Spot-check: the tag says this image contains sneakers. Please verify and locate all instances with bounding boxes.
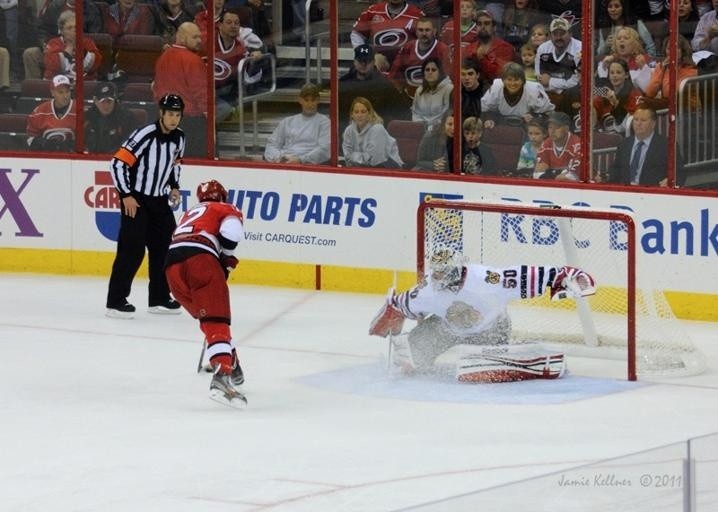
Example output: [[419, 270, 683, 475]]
[[394, 351, 432, 375], [229, 355, 245, 386], [209, 374, 247, 411]]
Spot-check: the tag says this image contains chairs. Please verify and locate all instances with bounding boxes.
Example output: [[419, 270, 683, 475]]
[[0, 33, 276, 161], [367, 45, 623, 183], [287, 0, 332, 92]]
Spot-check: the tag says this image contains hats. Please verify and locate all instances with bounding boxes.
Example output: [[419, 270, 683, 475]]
[[49, 73, 72, 90], [299, 82, 318, 99], [548, 17, 571, 32], [92, 81, 115, 103], [354, 44, 374, 60], [475, 10, 496, 20], [544, 111, 571, 127]]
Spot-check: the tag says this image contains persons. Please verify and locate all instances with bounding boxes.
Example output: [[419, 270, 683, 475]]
[[369, 251, 597, 383], [106, 94, 185, 313], [133, 0, 265, 95], [1, 0, 132, 151], [461, 1, 718, 194], [165, 180, 247, 410], [266, 1, 456, 174]]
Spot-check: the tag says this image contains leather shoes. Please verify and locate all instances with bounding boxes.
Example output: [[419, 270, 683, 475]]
[[147, 296, 180, 309], [105, 300, 135, 313]]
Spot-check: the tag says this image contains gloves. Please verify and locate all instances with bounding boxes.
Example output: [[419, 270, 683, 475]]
[[367, 288, 402, 338], [550, 261, 597, 302]]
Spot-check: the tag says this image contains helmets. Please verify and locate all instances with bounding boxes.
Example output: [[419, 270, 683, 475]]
[[196, 180, 227, 201], [158, 93, 184, 111], [426, 246, 465, 288]]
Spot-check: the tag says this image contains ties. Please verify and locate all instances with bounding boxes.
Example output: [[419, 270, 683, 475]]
[[628, 141, 644, 182]]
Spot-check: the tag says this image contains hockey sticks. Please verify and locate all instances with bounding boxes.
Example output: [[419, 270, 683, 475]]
[[197, 337, 209, 371]]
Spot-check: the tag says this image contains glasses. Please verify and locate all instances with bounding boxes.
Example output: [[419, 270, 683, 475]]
[[424, 67, 438, 72], [551, 30, 565, 35], [460, 71, 474, 76], [356, 58, 371, 64]]
